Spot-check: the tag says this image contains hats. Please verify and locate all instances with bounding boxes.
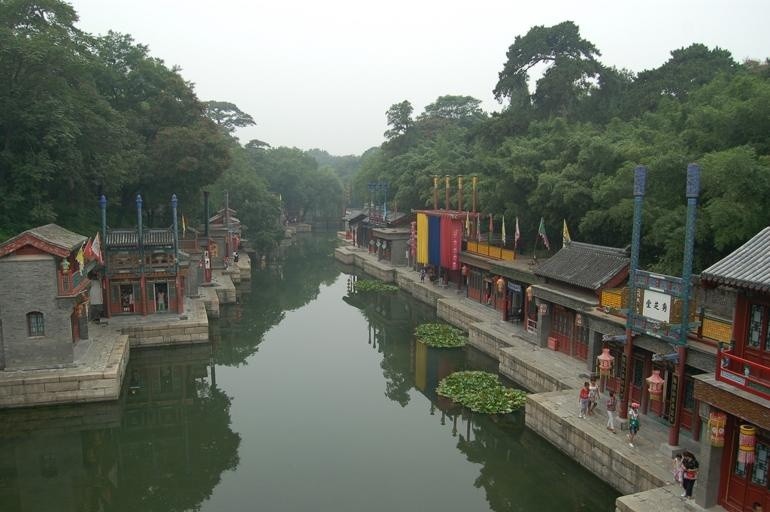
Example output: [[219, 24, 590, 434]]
[[631, 403, 641, 408]]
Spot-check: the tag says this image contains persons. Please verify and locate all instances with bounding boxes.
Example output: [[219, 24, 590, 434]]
[[578, 378, 601, 419], [628, 403, 640, 448], [418, 267, 449, 287], [672, 451, 700, 500], [74, 213, 240, 319], [605, 391, 617, 433]]
